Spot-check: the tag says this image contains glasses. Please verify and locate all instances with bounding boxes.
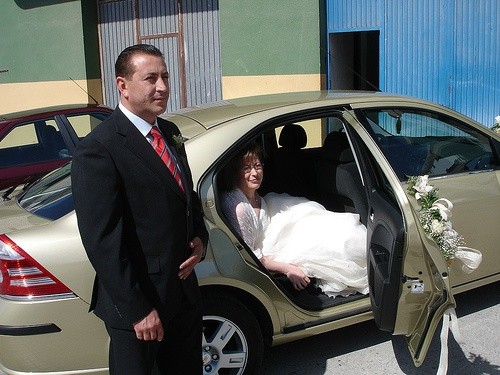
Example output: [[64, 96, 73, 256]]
[[241, 162, 264, 172]]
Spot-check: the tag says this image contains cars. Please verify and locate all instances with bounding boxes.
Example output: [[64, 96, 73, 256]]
[[0, 104, 116, 189], [1, 90, 500, 375]]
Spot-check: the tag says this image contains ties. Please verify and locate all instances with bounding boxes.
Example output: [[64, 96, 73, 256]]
[[149, 126, 185, 192]]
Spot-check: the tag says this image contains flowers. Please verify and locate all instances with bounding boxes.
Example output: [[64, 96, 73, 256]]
[[171, 132, 189, 151], [404, 173, 466, 262]]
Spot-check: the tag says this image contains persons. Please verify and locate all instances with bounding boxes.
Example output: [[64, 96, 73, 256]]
[[71, 44, 210, 375], [222, 140, 370, 300]]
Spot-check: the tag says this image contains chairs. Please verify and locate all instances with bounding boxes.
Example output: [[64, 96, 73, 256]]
[[269, 124, 368, 227], [34, 125, 64, 162]]
